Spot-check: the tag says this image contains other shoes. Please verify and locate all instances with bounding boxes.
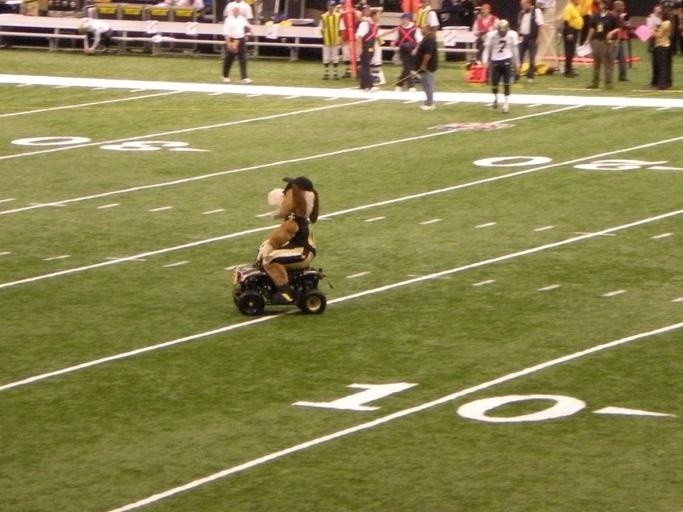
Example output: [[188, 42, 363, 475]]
[[562, 71, 578, 78], [586, 84, 598, 89], [409, 88, 416, 93], [528, 78, 533, 83], [510, 76, 515, 83], [605, 82, 611, 90], [647, 79, 673, 91], [420, 104, 436, 111], [333, 75, 339, 80], [394, 85, 402, 91], [493, 99, 497, 109], [502, 102, 508, 113], [341, 72, 351, 78], [323, 74, 330, 80], [354, 71, 380, 89], [619, 78, 628, 81]]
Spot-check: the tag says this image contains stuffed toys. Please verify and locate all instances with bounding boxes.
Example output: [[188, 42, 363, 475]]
[[255, 173, 319, 304]]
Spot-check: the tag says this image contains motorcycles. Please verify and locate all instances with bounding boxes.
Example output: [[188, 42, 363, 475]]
[[229, 249, 329, 317]]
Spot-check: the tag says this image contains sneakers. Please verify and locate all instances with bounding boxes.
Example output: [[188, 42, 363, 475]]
[[220, 76, 231, 83], [240, 78, 252, 84]]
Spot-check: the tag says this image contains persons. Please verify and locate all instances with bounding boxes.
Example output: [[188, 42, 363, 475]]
[[263, 12, 291, 57], [218, 6, 254, 84], [0, 0, 26, 48], [174, 0, 206, 12], [221, 0, 253, 19], [75, 16, 122, 56]]
